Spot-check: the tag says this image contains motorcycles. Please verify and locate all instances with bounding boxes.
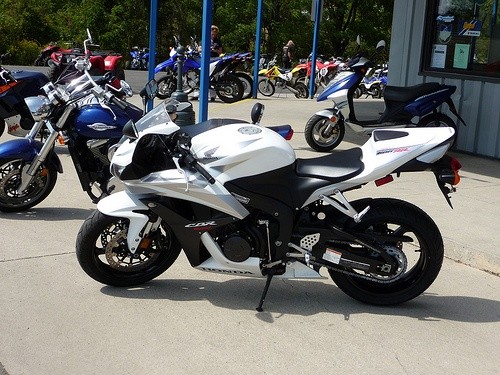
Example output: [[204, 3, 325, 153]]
[[0, 28, 294, 213], [304, 35, 466, 152], [34, 41, 128, 102], [74, 79, 463, 313], [153, 36, 389, 104], [129, 46, 149, 70], [0, 64, 69, 146]]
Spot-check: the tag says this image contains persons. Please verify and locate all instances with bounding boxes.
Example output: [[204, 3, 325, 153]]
[[190, 25, 222, 101], [283, 40, 295, 68]]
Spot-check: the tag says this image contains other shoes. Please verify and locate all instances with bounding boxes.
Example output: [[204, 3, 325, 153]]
[[189, 97, 199, 100], [211, 97, 215, 102]]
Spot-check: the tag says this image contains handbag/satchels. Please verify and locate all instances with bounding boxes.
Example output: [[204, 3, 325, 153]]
[[283, 56, 290, 66]]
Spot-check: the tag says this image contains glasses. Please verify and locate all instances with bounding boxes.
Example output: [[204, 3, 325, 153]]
[[211, 28, 218, 31]]
